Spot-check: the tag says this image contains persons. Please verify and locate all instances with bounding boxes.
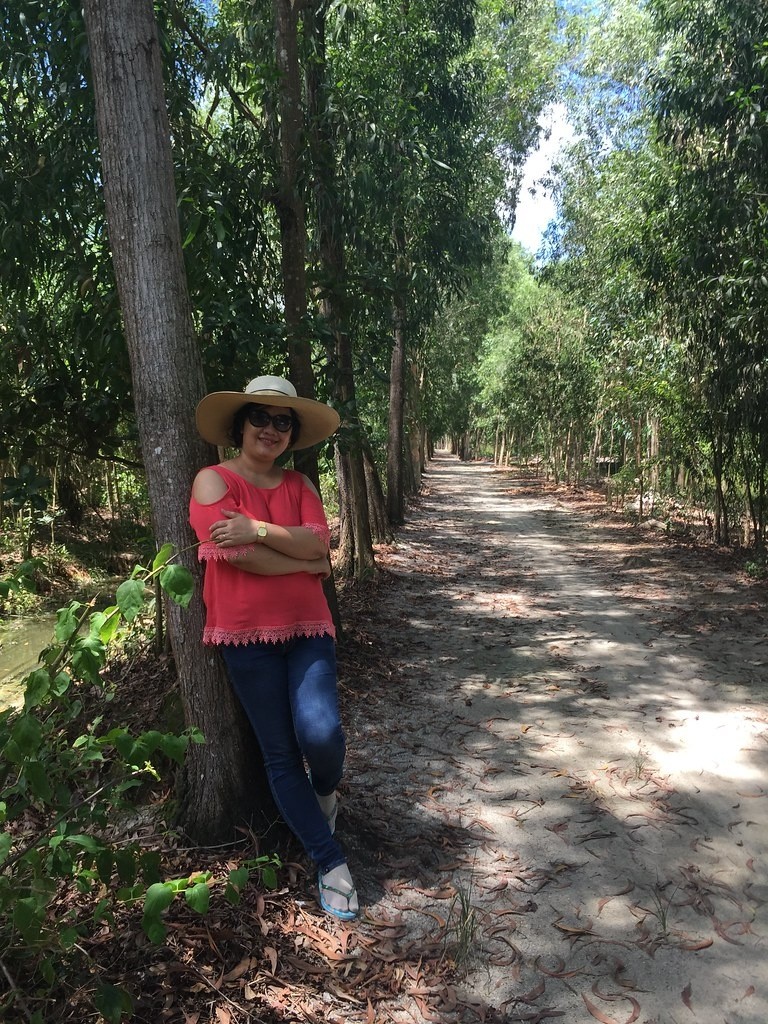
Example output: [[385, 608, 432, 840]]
[[190, 375, 359, 919]]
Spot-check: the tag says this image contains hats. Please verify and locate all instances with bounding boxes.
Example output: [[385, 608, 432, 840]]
[[195, 376, 340, 451]]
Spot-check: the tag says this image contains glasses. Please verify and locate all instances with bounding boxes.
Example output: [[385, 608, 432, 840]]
[[247, 409, 295, 433]]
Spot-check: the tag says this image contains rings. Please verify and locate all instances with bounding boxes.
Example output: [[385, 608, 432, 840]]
[[222, 534, 226, 540]]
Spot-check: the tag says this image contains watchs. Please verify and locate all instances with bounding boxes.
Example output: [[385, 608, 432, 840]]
[[256, 521, 267, 544]]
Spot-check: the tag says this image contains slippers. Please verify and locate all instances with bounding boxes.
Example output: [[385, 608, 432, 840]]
[[309, 768, 337, 835], [317, 869, 357, 919]]
